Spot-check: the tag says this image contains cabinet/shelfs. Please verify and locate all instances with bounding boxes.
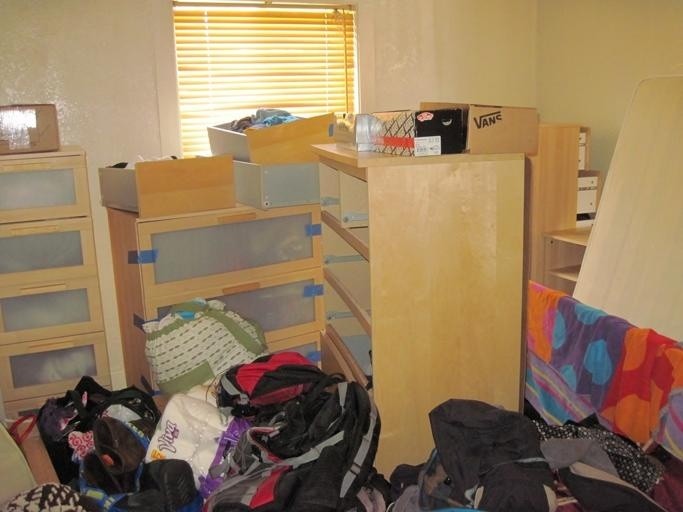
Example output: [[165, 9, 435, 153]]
[[105, 203, 323, 397], [525, 123, 603, 297], [0, 146, 128, 426], [312, 143, 526, 487]]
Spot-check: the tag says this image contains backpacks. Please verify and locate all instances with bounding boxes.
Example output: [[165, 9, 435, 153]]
[[2, 298, 668, 512]]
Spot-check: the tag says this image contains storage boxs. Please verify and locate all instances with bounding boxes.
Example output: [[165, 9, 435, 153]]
[[372, 109, 415, 139], [0, 103, 59, 154], [418, 100, 540, 156]]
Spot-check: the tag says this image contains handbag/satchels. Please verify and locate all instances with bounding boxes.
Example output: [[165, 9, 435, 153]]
[[142, 299, 268, 392]]
